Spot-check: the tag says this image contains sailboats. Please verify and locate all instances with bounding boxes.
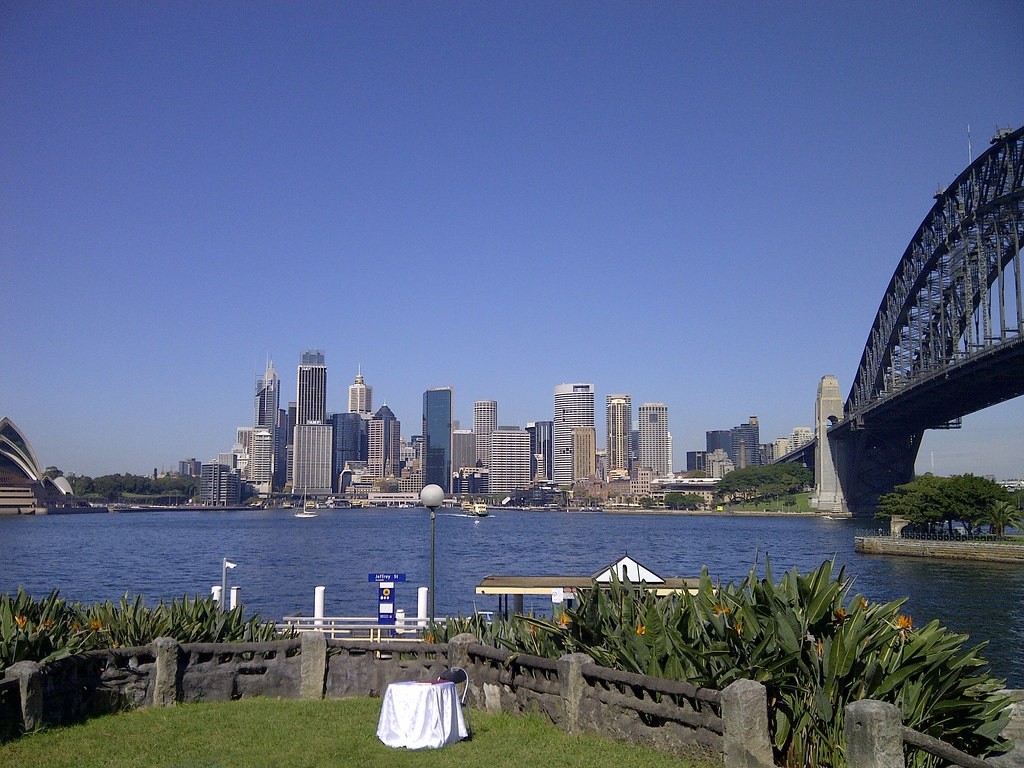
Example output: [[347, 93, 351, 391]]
[[294, 488, 317, 518]]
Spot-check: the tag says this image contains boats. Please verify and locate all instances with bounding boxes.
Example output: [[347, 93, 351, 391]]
[[467, 503, 489, 517], [305, 493, 353, 511], [281, 501, 294, 510]]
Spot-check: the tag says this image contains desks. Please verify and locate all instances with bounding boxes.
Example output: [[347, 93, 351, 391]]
[[376, 681, 471, 749]]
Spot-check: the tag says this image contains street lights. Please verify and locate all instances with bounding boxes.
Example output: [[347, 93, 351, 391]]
[[420, 484, 445, 644]]
